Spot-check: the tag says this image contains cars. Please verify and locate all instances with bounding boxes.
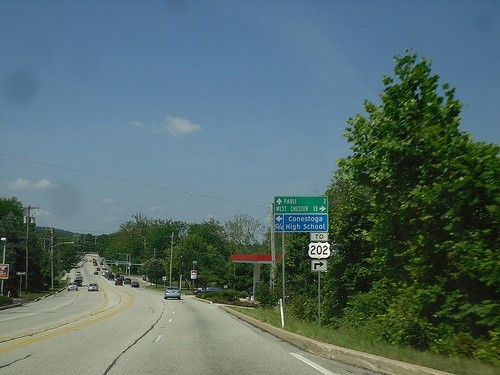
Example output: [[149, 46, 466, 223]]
[[89, 257, 121, 281], [76, 272, 81, 276], [131, 281, 139, 288], [88, 283, 98, 291], [123, 278, 131, 284], [75, 276, 83, 282], [67, 282, 79, 291], [163, 286, 181, 300], [70, 258, 88, 269], [74, 279, 83, 287], [115, 278, 123, 286]]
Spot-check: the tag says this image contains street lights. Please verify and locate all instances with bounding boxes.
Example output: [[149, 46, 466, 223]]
[[50, 226, 75, 290], [1, 236, 8, 296]]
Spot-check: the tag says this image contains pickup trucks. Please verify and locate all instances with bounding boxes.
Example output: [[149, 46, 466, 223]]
[[194, 287, 223, 296]]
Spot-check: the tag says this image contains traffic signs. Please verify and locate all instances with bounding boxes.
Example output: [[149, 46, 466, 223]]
[[274, 215, 328, 232], [274, 195, 328, 214]]
